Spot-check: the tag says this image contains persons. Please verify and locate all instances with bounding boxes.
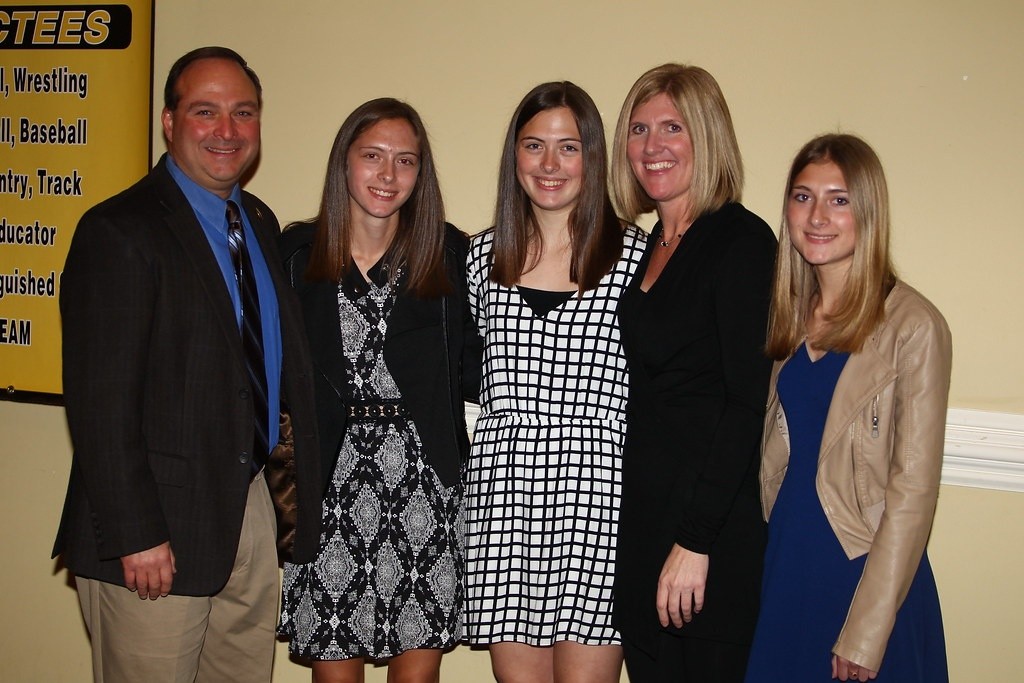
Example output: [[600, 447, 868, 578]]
[[745, 133, 955, 683], [612, 62, 780, 683], [265, 96, 486, 683], [462, 80, 651, 683], [50, 43, 323, 683]]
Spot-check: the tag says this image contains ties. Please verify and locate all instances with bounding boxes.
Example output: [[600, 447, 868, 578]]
[[226, 198, 270, 484]]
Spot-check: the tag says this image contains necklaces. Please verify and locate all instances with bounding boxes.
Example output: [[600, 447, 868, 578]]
[[657, 223, 686, 248]]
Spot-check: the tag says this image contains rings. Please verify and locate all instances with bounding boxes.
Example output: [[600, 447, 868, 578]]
[[849, 671, 858, 677]]
